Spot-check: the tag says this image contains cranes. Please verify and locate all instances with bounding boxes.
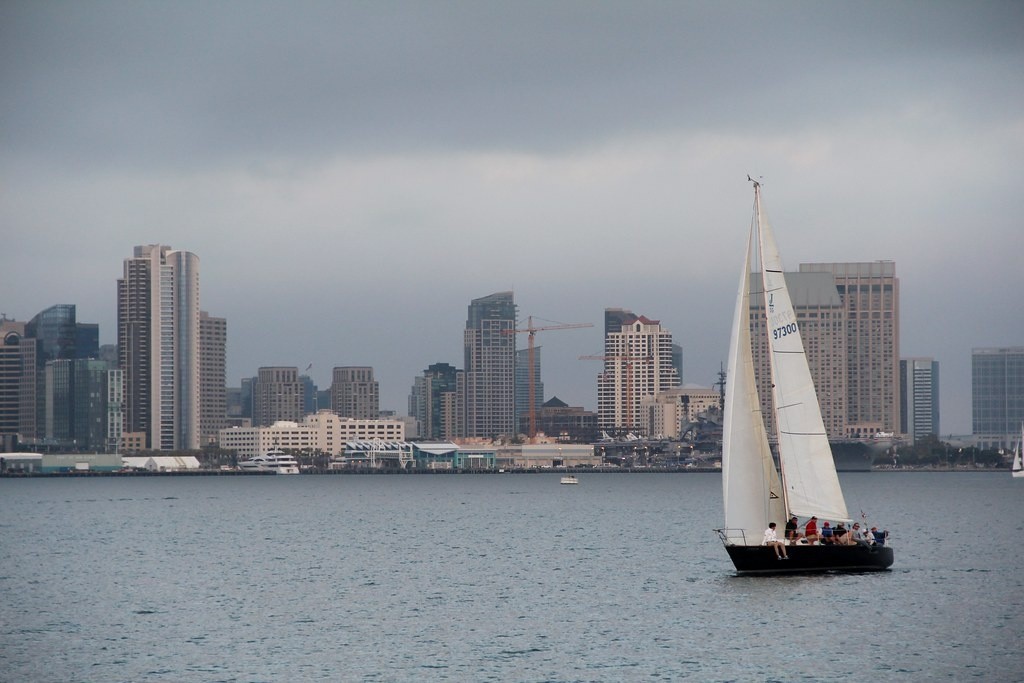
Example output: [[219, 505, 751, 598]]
[[498, 315, 595, 445], [578, 332, 651, 433]]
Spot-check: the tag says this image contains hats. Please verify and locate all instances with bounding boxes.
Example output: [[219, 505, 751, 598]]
[[812, 516, 817, 519], [863, 529, 868, 534], [871, 527, 877, 530], [824, 522, 829, 526]]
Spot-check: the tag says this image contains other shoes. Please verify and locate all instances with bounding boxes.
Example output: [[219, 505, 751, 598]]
[[790, 541, 797, 546], [778, 556, 783, 560], [783, 555, 789, 559]]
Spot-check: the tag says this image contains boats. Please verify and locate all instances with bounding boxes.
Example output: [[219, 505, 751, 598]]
[[237, 450, 301, 475], [560, 475, 578, 484]]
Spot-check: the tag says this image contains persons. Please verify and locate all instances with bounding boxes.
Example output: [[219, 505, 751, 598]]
[[763, 523, 789, 560], [785, 517, 803, 545], [820, 521, 884, 545], [806, 516, 823, 545]]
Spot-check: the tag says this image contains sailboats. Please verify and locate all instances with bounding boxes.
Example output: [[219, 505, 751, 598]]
[[710, 172, 895, 575], [1011, 419, 1024, 478]]
